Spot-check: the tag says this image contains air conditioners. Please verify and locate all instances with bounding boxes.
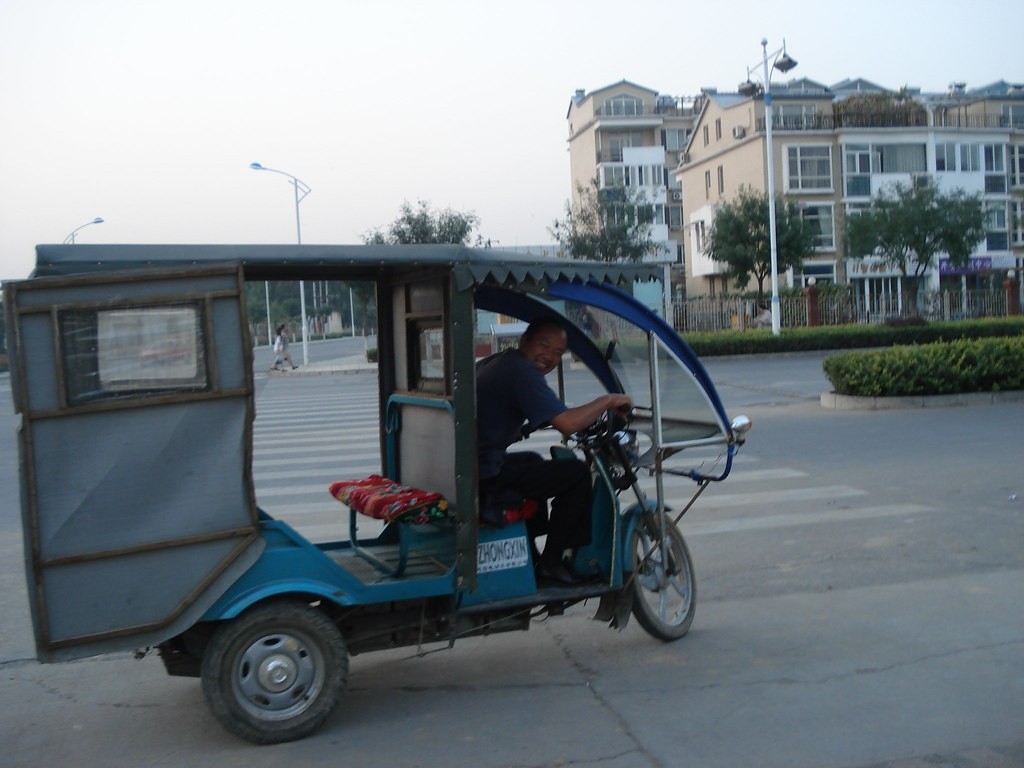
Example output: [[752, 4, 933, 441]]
[[673, 192, 681, 201], [680, 152, 688, 164], [732, 125, 743, 138], [914, 172, 933, 192]]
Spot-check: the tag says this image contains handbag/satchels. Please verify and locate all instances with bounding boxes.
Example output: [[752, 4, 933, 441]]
[[274, 354, 283, 364]]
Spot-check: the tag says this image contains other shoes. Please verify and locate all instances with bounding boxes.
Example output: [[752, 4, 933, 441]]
[[292, 366, 299, 370]]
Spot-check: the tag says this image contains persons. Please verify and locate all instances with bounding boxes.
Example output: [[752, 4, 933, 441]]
[[752, 306, 772, 325], [728, 310, 740, 329], [475, 319, 631, 583], [271, 325, 299, 371]]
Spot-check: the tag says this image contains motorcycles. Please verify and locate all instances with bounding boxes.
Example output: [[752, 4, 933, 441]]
[[2, 242, 754, 747]]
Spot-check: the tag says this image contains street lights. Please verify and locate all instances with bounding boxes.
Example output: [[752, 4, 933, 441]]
[[735, 37, 798, 335], [247, 162, 313, 368], [64, 215, 105, 242]]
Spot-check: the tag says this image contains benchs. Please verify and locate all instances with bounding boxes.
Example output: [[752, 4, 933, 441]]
[[329, 473, 458, 576], [481, 485, 544, 524]]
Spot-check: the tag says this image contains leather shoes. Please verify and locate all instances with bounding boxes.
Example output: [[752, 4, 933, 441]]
[[536, 563, 601, 588]]
